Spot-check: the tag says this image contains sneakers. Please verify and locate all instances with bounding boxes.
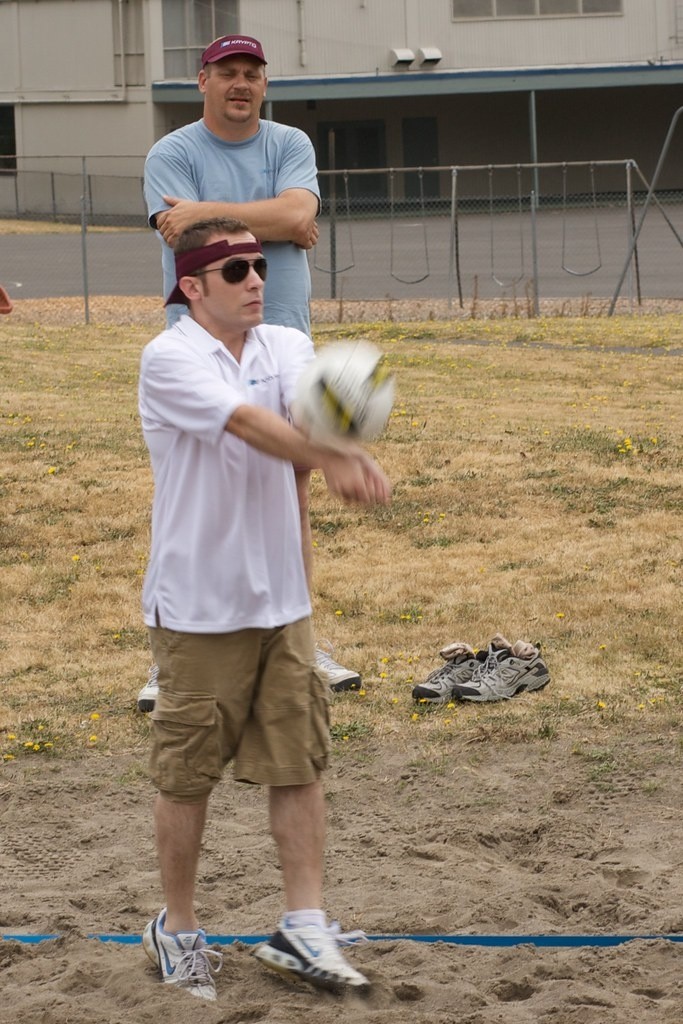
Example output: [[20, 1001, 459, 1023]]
[[452, 641, 550, 702], [142, 907, 223, 1000], [315, 638, 361, 692], [137, 663, 160, 712], [254, 922, 372, 996], [412, 650, 489, 704]]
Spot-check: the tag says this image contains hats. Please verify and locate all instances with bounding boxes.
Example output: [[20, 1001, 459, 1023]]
[[163, 238, 263, 308], [201, 35, 267, 69]]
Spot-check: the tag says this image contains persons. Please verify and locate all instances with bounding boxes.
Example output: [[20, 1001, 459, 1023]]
[[136, 217, 390, 1002], [137, 34, 363, 711]]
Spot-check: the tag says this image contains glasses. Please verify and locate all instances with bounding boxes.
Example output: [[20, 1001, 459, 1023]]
[[190, 259, 267, 282]]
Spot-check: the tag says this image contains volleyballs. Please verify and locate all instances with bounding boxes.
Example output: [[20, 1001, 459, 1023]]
[[296, 339, 402, 446]]
